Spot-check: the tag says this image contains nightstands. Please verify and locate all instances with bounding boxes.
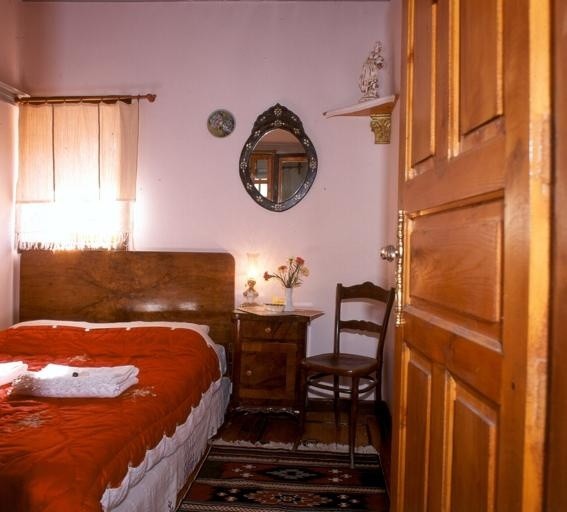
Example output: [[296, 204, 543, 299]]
[[232, 304, 326, 428]]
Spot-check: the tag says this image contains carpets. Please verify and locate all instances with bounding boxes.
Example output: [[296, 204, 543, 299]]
[[167, 438, 390, 512]]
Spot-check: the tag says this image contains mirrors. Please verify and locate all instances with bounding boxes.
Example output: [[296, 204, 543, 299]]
[[239, 103, 318, 212]]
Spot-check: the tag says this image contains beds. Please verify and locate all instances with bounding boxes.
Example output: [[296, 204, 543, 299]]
[[0, 248, 237, 511]]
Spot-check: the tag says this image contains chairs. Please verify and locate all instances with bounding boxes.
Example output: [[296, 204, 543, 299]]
[[297, 280, 396, 470]]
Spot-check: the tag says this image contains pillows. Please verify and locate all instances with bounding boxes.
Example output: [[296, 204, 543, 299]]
[[7, 319, 210, 356]]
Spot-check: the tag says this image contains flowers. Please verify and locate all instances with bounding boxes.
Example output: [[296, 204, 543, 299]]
[[264, 256, 309, 288]]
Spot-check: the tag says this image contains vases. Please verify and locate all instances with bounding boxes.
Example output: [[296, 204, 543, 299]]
[[283, 288, 295, 311]]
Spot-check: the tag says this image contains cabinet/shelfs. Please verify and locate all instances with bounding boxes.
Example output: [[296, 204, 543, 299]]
[[248, 150, 308, 205]]
